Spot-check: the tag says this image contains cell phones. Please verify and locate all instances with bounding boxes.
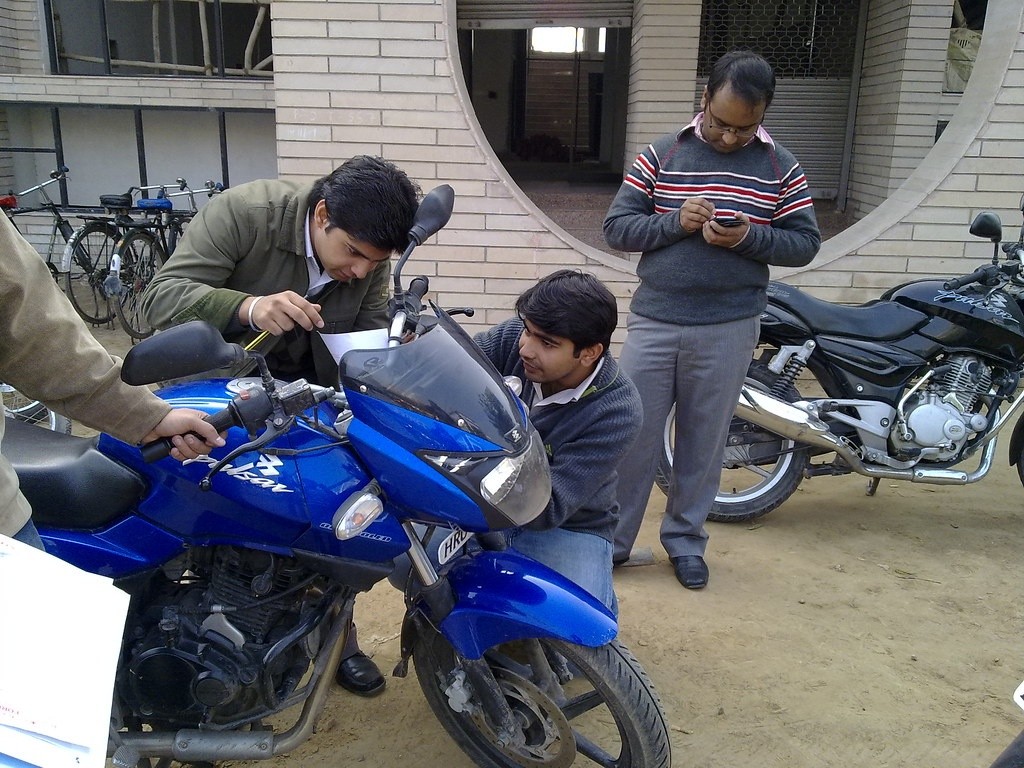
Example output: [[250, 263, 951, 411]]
[[713, 218, 744, 227]]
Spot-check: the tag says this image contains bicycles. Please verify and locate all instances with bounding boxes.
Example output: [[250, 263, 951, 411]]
[[105, 181, 227, 346], [1, 165, 107, 305], [63, 176, 188, 333]]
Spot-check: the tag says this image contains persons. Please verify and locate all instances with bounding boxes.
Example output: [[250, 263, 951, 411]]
[[0, 203, 226, 551], [139, 155, 419, 698], [601, 49, 821, 593], [472, 268, 646, 558]]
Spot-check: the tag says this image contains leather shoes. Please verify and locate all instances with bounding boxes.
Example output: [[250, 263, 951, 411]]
[[669, 555, 709, 589], [334, 653, 387, 697]]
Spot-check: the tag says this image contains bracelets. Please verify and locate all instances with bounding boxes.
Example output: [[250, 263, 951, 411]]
[[248, 296, 266, 332]]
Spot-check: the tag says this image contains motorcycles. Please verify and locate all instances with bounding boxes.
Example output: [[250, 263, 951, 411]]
[[644, 195, 1024, 525], [2, 183, 676, 768]]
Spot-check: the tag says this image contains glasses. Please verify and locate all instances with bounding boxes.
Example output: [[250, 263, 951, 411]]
[[709, 99, 765, 138]]
